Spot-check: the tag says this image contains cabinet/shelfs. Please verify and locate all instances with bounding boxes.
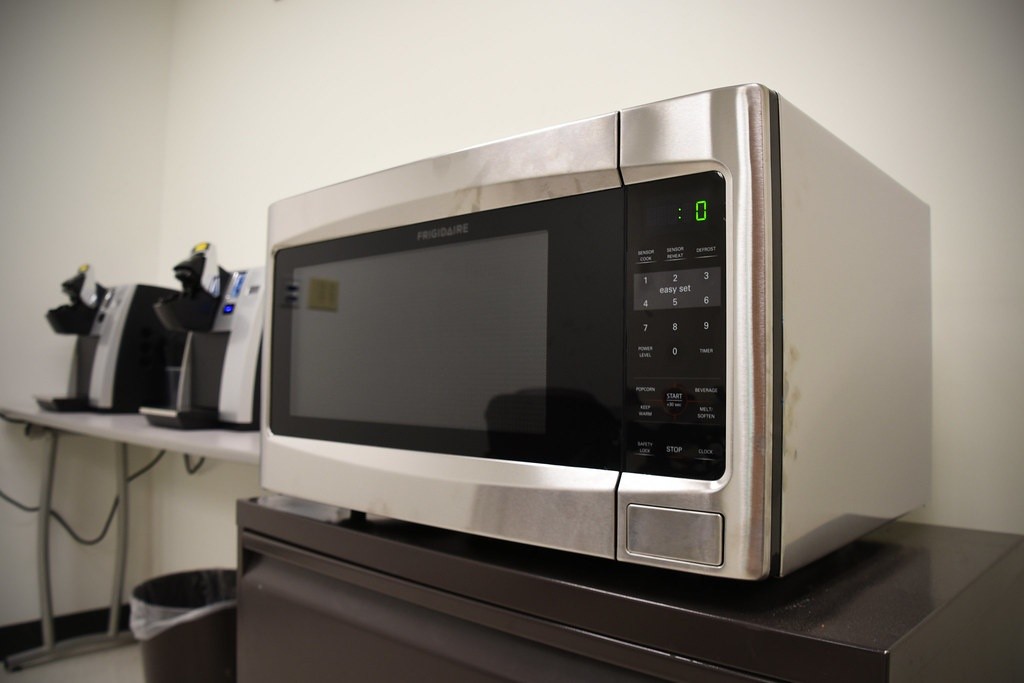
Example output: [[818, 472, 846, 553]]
[[234, 495, 1024, 683]]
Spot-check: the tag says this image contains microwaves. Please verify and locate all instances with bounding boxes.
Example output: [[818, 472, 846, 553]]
[[261, 83, 930, 579]]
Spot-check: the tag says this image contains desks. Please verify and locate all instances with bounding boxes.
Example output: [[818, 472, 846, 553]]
[[0, 407, 261, 674]]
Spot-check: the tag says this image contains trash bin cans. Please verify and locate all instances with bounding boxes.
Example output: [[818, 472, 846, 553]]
[[130, 567, 237, 682]]
